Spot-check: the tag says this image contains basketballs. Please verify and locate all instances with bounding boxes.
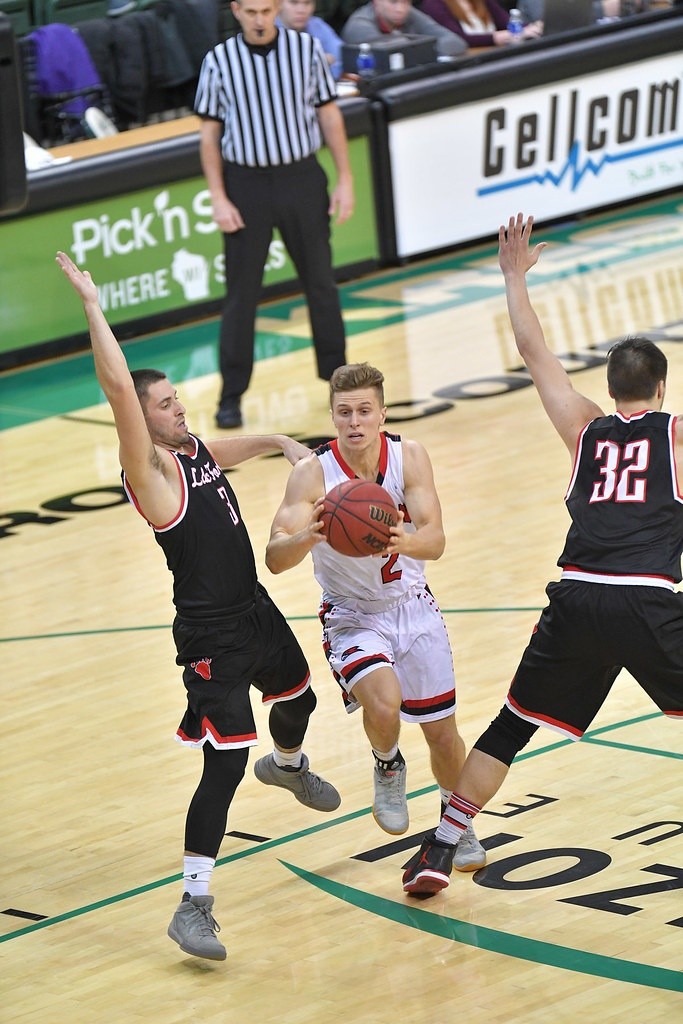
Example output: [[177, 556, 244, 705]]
[[317, 479, 398, 557]]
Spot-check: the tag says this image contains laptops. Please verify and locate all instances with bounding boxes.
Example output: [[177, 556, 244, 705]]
[[540, 0, 595, 37]]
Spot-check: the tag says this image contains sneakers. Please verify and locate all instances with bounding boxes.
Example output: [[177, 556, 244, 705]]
[[438, 801, 486, 870], [371, 754, 409, 835], [211, 397, 243, 427], [167, 895, 226, 960], [253, 752, 341, 811], [403, 833, 458, 893]]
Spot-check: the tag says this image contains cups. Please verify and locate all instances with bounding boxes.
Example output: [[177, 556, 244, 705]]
[[389, 51, 403, 72]]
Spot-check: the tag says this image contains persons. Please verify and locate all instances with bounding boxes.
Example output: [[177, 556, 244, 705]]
[[190, 1, 355, 429], [275, 1, 350, 85], [54, 249, 342, 962], [264, 363, 486, 874], [416, 0, 545, 54], [404, 213, 683, 899], [340, 0, 471, 83]]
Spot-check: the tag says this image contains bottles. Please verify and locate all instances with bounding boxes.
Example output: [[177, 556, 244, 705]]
[[506, 9, 524, 45], [358, 43, 374, 76]]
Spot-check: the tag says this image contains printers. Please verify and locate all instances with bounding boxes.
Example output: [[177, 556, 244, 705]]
[[341, 32, 438, 75]]
